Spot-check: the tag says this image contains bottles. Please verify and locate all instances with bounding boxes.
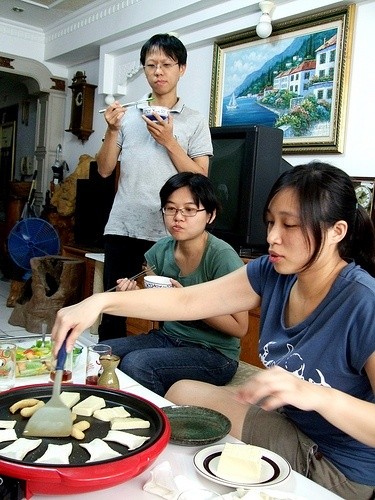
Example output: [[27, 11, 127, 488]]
[[99, 355, 122, 390]]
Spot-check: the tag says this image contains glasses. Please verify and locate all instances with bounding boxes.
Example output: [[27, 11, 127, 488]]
[[163, 208, 205, 217], [144, 62, 179, 69]]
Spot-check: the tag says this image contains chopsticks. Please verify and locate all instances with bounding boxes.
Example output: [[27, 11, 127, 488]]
[[98, 97, 155, 114], [104, 263, 157, 292]]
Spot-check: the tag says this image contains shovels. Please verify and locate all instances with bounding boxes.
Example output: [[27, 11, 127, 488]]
[[20, 330, 72, 437]]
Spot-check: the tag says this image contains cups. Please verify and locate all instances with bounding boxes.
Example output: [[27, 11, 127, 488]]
[[86, 344, 112, 384], [1, 343, 19, 381]]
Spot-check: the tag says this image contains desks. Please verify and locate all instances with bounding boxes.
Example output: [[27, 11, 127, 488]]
[[62, 245, 268, 372]]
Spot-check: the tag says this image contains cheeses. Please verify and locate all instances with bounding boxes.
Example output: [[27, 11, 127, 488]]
[[215, 442, 264, 483]]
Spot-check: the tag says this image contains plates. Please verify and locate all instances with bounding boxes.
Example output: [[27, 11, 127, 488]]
[[192, 442, 293, 488], [0, 333, 83, 375], [159, 403, 232, 446]]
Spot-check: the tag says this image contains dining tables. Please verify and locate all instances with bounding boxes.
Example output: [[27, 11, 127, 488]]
[[0, 336, 344, 500]]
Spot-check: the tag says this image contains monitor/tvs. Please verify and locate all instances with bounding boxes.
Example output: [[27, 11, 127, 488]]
[[208, 125, 294, 258]]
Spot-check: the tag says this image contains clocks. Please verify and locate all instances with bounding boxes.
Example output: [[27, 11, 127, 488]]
[[351, 177, 375, 222], [65, 71, 98, 144]]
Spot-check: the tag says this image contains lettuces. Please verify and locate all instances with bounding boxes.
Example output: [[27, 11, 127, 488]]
[[16, 339, 82, 375]]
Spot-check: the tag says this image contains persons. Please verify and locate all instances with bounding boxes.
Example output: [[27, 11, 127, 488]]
[[50, 160, 375, 499], [97, 33, 211, 336], [97, 172, 249, 398]]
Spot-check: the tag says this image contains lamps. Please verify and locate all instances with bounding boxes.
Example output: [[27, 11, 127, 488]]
[[256, 1, 274, 38]]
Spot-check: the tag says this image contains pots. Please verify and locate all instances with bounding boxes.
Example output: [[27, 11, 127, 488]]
[[0, 382, 171, 494]]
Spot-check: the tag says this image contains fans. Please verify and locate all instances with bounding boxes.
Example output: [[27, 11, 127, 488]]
[[8, 218, 60, 280]]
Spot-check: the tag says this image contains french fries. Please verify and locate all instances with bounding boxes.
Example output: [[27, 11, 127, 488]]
[[9, 399, 91, 439]]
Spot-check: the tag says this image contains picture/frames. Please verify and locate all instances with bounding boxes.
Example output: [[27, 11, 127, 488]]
[[208, 2, 356, 153]]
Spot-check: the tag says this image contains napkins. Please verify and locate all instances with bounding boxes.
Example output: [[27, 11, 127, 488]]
[[143, 460, 188, 500]]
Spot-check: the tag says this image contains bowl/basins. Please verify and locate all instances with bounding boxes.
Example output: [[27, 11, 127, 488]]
[[142, 107, 170, 120], [145, 276, 174, 290]]
